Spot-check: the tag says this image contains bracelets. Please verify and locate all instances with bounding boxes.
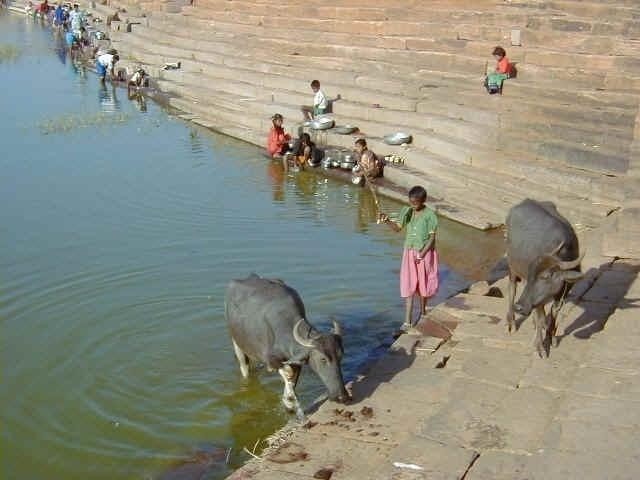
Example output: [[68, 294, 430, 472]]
[[383, 216, 388, 222], [417, 255, 422, 260]]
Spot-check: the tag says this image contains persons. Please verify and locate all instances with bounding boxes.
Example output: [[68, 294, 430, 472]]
[[293, 133, 322, 172], [300, 79, 328, 121], [127, 91, 148, 114], [265, 159, 287, 204], [267, 113, 291, 160], [97, 89, 122, 118], [0, 0, 146, 91], [378, 185, 439, 333], [353, 188, 382, 238], [295, 170, 327, 225], [352, 139, 380, 178], [485, 46, 513, 93], [282, 133, 310, 174]]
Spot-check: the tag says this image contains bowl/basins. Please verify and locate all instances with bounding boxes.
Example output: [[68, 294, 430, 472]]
[[383, 131, 412, 145], [334, 125, 360, 134], [309, 119, 334, 130], [338, 151, 361, 162]]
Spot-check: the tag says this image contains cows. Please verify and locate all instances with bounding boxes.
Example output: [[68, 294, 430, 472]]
[[223, 270, 350, 430], [504, 197, 587, 360]]
